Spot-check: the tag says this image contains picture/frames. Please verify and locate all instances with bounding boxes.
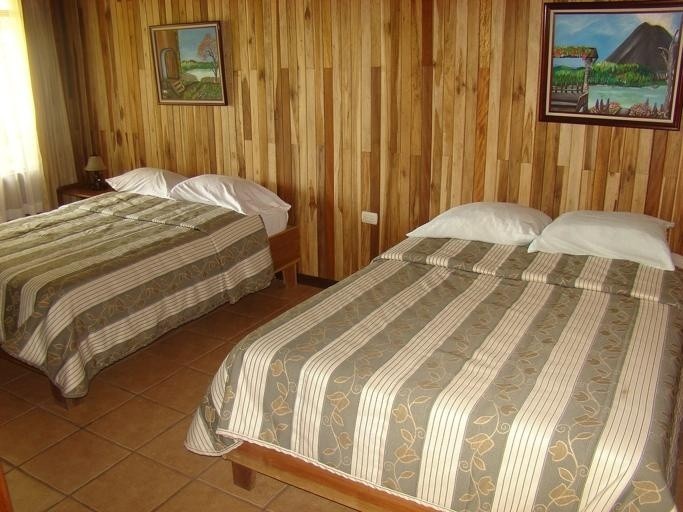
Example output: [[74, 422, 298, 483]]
[[538, 0, 683, 131], [148, 21, 227, 106]]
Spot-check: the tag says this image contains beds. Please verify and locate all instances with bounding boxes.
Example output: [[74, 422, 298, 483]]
[[183, 237, 683, 512], [0, 190, 301, 399]]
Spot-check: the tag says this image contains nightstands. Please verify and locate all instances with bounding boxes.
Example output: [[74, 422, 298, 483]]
[[56, 182, 115, 207]]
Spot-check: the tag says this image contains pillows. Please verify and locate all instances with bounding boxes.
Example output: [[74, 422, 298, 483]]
[[405, 201, 553, 247], [169, 174, 292, 238], [526, 210, 676, 275], [104, 166, 190, 201]]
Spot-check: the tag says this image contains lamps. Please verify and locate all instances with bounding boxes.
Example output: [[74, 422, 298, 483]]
[[82, 155, 106, 191]]
